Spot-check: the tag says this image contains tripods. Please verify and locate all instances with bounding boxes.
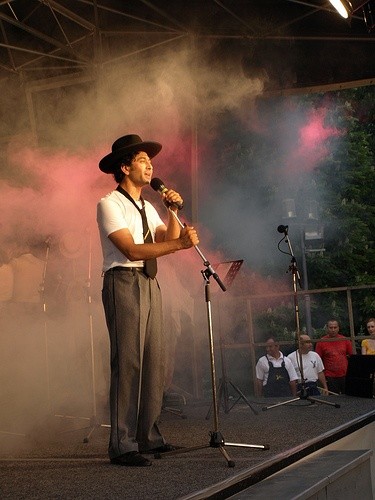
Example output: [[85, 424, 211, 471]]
[[155, 204, 346, 466], [0, 238, 112, 443]]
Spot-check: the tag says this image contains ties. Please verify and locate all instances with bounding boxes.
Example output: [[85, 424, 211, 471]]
[[116, 184, 158, 280]]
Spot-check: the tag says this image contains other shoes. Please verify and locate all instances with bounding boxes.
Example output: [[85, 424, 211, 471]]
[[110, 452, 153, 467], [140, 445, 176, 454]]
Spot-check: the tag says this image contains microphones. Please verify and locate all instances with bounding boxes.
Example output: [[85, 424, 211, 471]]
[[150, 178, 183, 210], [278, 225, 302, 289], [43, 235, 53, 244]]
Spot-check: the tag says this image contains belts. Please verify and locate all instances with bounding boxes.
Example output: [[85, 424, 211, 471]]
[[112, 266, 145, 273]]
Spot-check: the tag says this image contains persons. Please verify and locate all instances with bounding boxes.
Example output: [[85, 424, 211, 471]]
[[361, 317, 375, 355], [96, 134, 200, 467], [286, 335, 329, 396], [256, 336, 299, 398], [314, 319, 357, 395]]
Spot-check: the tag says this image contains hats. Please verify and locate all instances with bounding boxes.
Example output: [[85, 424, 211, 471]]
[[98, 134, 162, 174]]
